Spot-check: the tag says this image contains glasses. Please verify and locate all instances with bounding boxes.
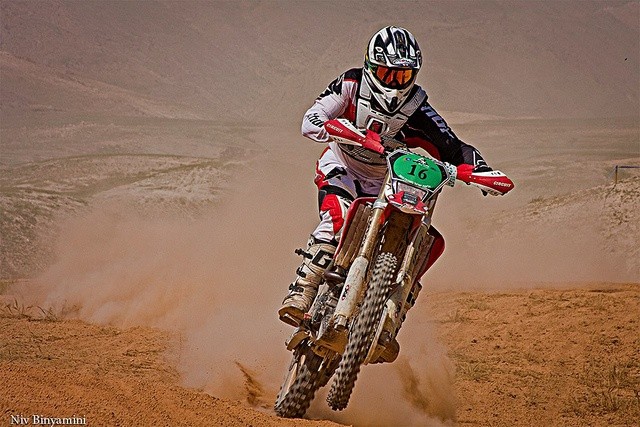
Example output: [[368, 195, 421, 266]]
[[370, 65, 415, 87]]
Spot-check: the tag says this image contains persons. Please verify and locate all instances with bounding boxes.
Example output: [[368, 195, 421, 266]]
[[279, 25, 495, 364]]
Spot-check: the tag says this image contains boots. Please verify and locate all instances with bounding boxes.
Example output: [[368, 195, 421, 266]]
[[279, 234, 338, 328], [377, 283, 423, 362]]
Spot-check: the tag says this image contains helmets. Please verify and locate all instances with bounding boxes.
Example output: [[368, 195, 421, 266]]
[[364, 26, 422, 114]]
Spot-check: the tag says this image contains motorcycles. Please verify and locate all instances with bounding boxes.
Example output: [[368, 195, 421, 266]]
[[274, 114, 515, 418]]
